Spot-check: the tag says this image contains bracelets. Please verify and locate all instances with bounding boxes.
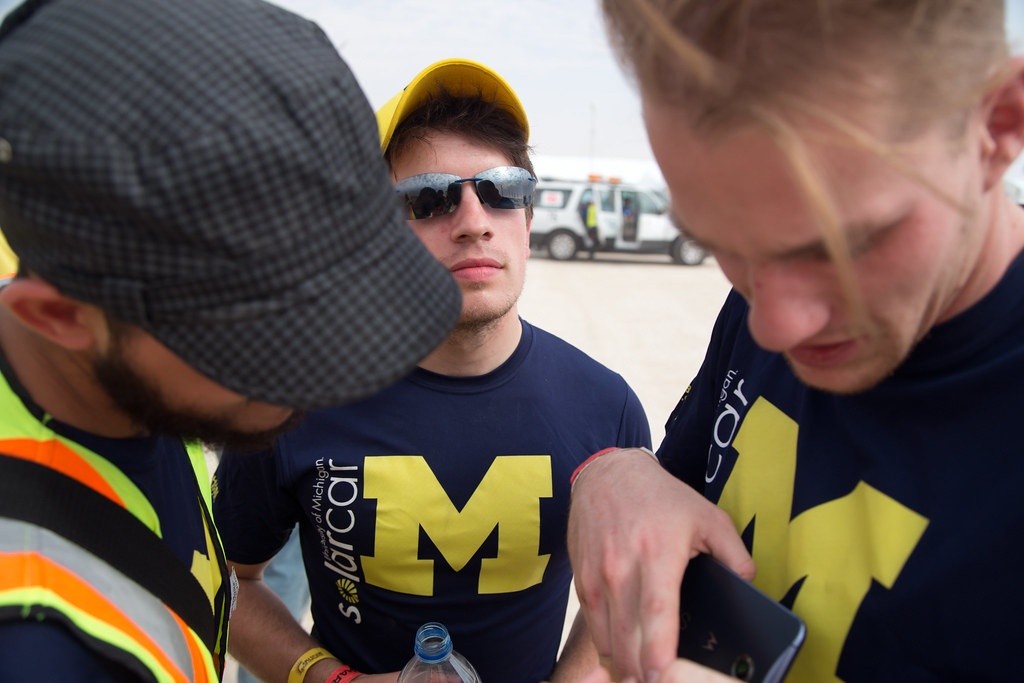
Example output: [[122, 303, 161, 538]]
[[571, 445, 660, 493], [325, 665, 363, 683], [287, 647, 336, 683]]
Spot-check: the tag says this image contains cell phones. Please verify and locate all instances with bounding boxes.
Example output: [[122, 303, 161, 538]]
[[676, 552, 807, 683]]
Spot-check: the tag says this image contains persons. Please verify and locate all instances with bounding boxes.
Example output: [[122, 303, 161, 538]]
[[211, 62, 653, 683], [580, 192, 602, 260], [565, 2, 1024, 683], [0, 0, 465, 683], [620, 195, 637, 217]]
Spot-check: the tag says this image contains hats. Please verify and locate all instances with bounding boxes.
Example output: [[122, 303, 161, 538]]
[[373, 58, 530, 158], [0, 0, 461, 409]]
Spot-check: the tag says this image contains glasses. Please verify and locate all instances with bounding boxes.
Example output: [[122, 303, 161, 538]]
[[395, 165, 538, 220]]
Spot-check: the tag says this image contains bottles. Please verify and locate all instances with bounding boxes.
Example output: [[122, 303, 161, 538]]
[[397, 621, 482, 683]]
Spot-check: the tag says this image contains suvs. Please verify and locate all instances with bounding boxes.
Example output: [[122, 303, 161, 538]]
[[529, 173, 709, 265]]
